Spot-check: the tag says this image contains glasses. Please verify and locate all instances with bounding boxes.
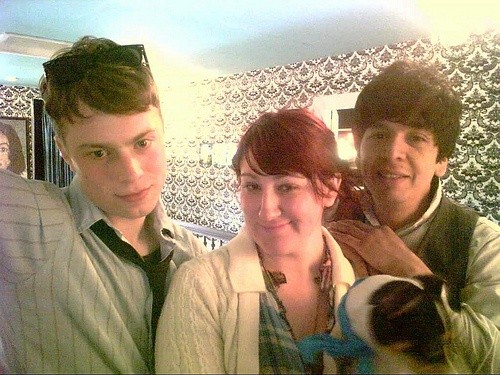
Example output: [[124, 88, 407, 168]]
[[42, 44, 151, 86]]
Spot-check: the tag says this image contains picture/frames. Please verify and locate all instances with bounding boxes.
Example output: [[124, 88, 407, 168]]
[[0, 116, 33, 179]]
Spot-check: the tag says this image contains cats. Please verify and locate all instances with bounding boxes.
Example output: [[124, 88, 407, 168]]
[[343, 271, 472, 375]]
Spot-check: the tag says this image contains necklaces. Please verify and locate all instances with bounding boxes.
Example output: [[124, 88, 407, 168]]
[[255, 242, 326, 338]]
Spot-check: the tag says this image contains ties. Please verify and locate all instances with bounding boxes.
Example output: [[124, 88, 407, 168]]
[[89, 219, 175, 375]]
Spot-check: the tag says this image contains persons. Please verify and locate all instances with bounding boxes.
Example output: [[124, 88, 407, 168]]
[[0, 35, 211, 375], [323, 57, 500, 375], [151, 105, 385, 375]]
